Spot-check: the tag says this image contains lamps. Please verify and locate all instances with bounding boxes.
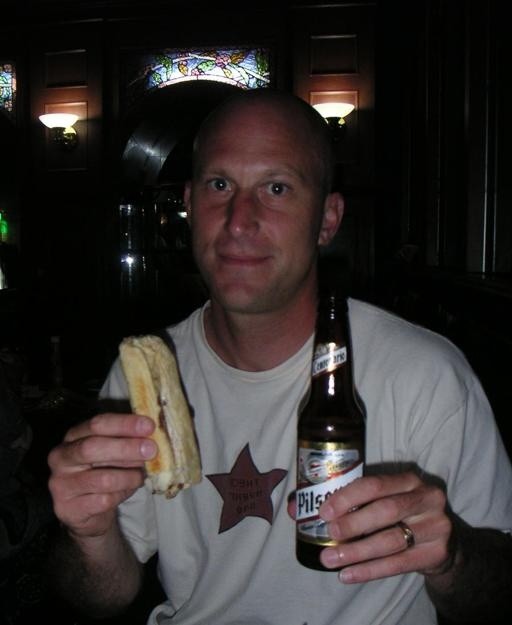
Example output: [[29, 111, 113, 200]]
[[40, 112, 79, 146], [312, 102, 355, 131]]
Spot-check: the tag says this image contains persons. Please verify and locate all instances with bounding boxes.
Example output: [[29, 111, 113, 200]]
[[47, 86, 512, 625]]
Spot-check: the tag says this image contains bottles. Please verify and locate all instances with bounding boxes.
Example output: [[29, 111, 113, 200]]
[[292, 289, 370, 576]]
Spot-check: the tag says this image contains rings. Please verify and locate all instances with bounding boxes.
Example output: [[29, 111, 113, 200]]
[[399, 521, 417, 552]]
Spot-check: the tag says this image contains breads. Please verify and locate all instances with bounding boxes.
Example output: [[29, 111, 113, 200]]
[[120, 335, 202, 499]]
